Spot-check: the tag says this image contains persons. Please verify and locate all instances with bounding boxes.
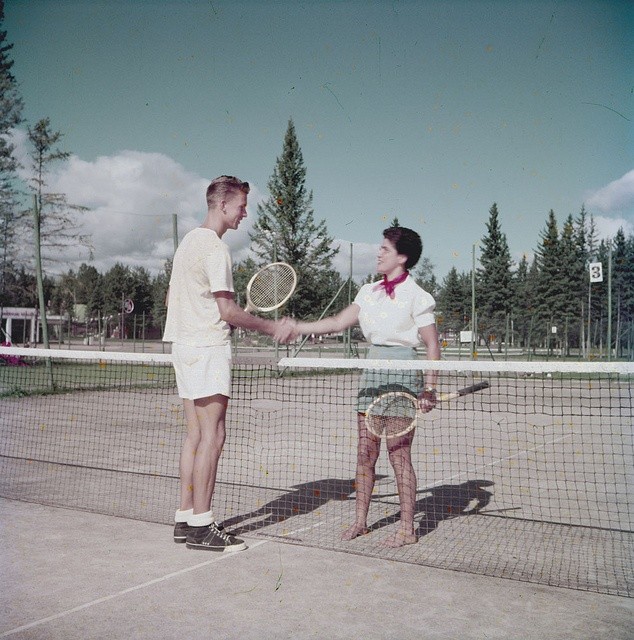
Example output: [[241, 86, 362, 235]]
[[274, 227, 440, 547], [163, 175, 300, 550]]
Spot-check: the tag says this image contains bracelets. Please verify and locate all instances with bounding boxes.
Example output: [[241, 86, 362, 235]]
[[424, 387, 437, 394]]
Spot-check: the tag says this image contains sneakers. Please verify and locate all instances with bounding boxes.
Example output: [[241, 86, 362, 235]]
[[186, 512, 246, 552], [174, 509, 193, 542]]
